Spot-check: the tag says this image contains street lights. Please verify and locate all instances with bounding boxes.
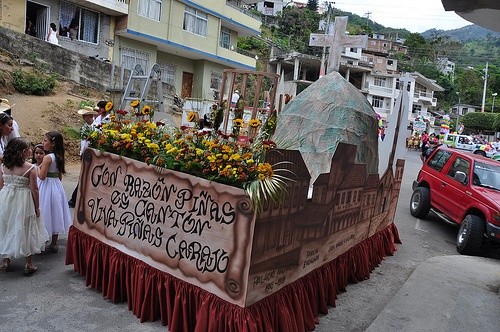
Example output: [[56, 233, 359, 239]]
[[490, 93, 499, 113]]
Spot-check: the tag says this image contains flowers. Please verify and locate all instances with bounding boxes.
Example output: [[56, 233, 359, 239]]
[[77, 98, 299, 214]]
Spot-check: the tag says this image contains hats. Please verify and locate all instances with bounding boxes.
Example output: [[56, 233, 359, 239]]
[[78, 106, 99, 115], [0, 98, 15, 112]]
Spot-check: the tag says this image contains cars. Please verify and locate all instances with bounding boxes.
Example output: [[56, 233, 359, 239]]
[[441, 134, 500, 164]]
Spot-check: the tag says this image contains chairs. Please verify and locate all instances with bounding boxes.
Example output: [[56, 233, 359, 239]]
[[454, 161, 468, 174]]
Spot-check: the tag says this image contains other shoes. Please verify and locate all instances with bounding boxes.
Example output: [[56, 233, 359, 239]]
[[25, 263, 39, 274], [0, 259, 10, 271], [68, 198, 76, 208], [43, 245, 58, 253]]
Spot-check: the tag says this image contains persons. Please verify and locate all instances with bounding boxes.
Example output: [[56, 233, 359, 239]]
[[203, 105, 217, 127], [0, 98, 40, 277], [377, 125, 389, 141], [231, 90, 239, 112], [25, 17, 38, 36], [460, 137, 467, 144], [68, 100, 112, 208], [408, 129, 440, 156], [495, 138, 500, 154], [47, 23, 60, 45], [470, 133, 486, 144], [60, 25, 83, 40], [31, 131, 73, 256]]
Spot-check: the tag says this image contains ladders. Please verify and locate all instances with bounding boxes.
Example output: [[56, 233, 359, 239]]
[[119, 63, 165, 113]]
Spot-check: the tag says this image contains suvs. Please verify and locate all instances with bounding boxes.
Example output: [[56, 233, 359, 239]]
[[409, 145, 500, 257]]
